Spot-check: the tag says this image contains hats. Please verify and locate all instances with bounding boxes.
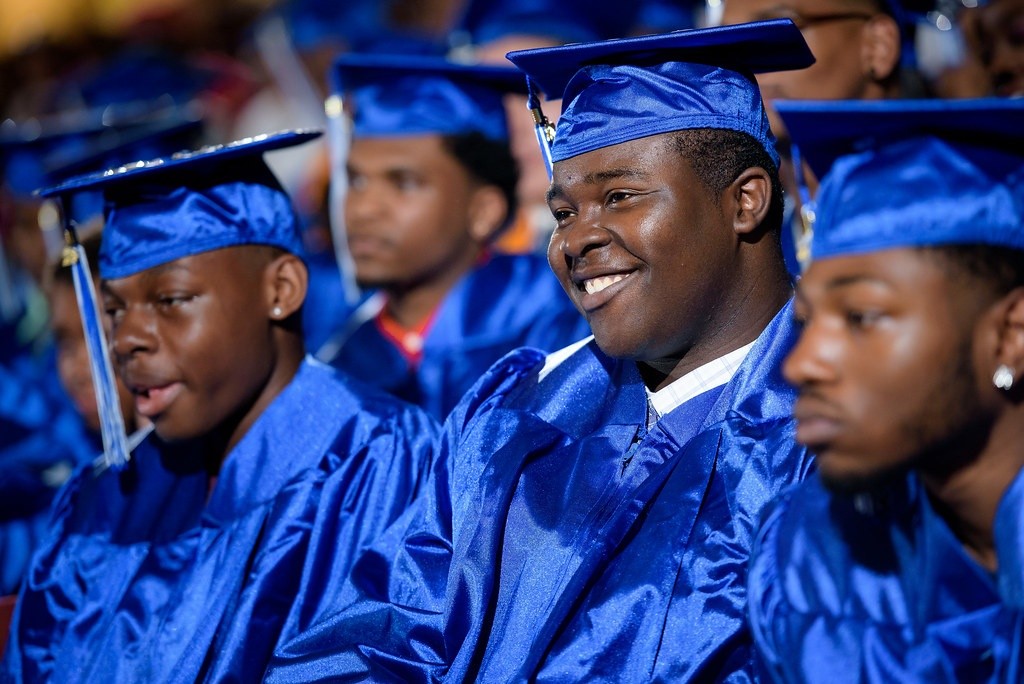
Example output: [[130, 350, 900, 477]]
[[325, 54, 542, 305], [772, 97, 1024, 256], [505, 19, 817, 178], [32, 129, 328, 469]]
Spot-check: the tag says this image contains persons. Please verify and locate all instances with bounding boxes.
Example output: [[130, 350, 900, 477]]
[[0, 0, 443, 684], [312, 0, 1024, 684]]
